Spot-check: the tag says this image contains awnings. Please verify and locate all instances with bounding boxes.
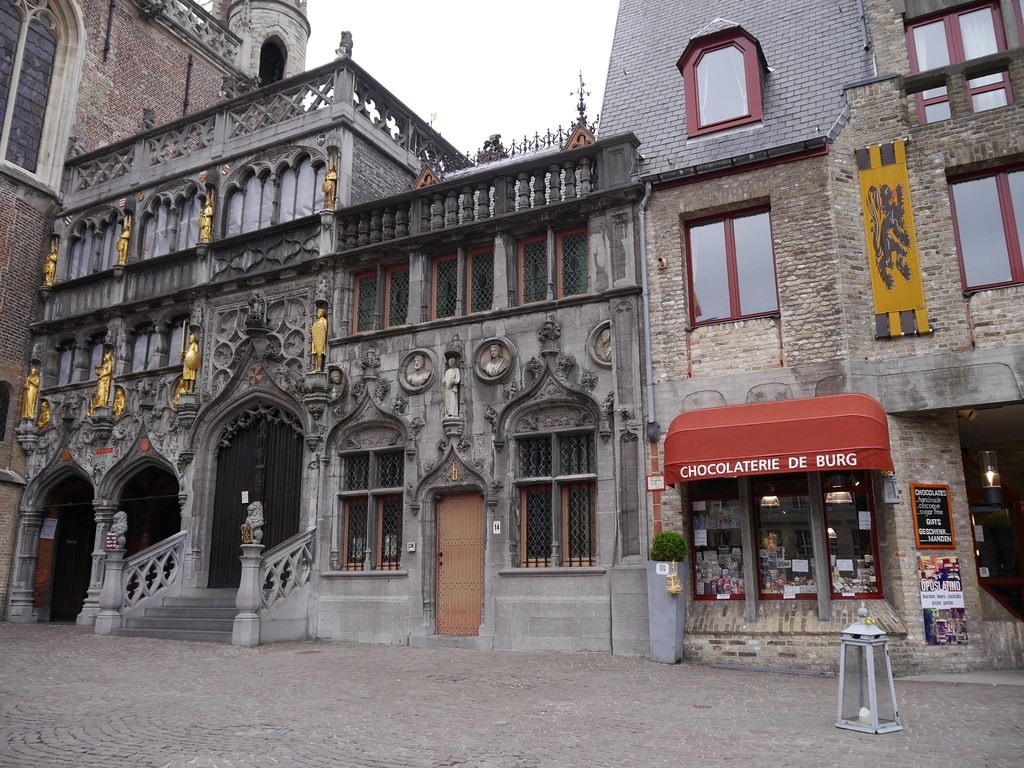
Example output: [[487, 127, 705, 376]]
[[664, 394, 895, 478]]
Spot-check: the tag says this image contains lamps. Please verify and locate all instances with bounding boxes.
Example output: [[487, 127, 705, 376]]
[[826, 492, 853, 503], [965, 450, 1004, 513], [760, 496, 780, 506]]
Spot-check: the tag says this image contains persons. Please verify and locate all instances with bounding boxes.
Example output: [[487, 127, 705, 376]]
[[482, 345, 509, 376], [180, 333, 201, 392], [37, 402, 51, 429], [92, 351, 112, 408], [310, 308, 327, 372], [116, 216, 129, 265], [43, 241, 57, 286], [113, 389, 125, 419], [20, 367, 39, 420], [174, 380, 185, 404], [322, 162, 336, 209], [199, 192, 213, 241], [441, 358, 461, 416], [331, 371, 340, 399], [595, 328, 612, 362], [408, 355, 431, 387]]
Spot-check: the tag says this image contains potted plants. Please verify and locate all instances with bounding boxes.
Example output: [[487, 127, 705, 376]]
[[647, 531, 688, 664]]
